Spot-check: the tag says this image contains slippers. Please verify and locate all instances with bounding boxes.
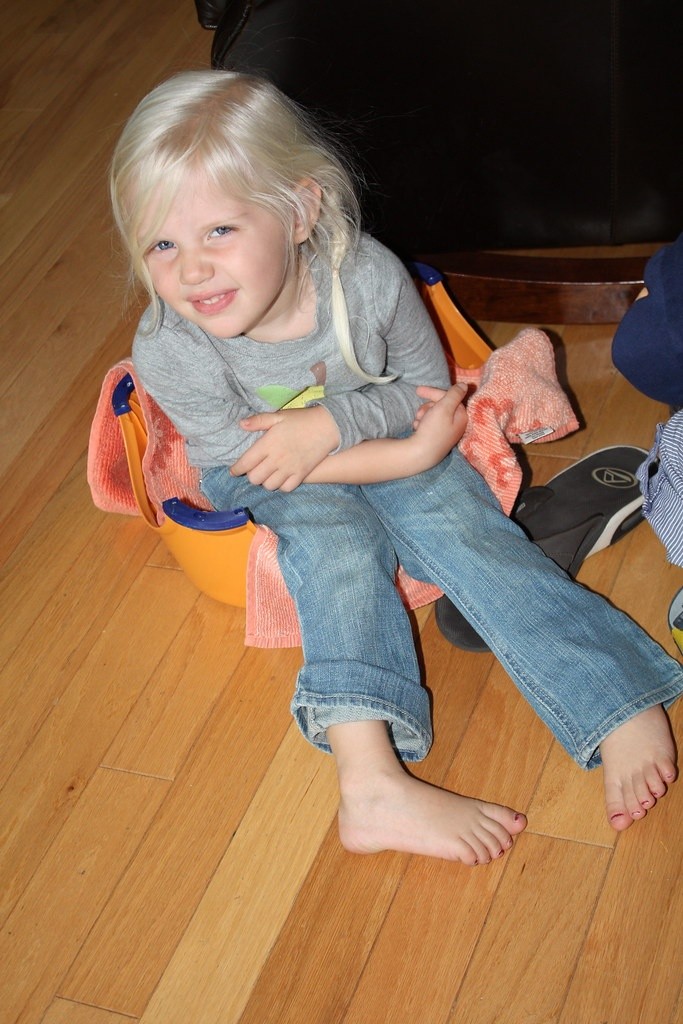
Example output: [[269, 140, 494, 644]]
[[667, 586, 683, 656], [433, 443, 660, 650]]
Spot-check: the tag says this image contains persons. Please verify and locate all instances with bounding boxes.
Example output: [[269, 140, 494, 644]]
[[111, 70, 683, 865]]
